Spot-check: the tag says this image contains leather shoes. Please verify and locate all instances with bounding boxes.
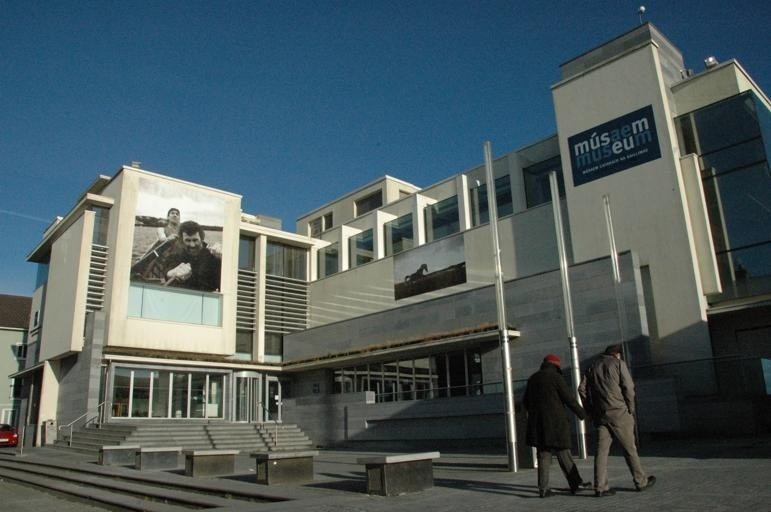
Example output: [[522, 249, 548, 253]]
[[575, 482, 592, 492], [595, 488, 617, 497], [636, 476, 656, 492], [540, 490, 555, 498]]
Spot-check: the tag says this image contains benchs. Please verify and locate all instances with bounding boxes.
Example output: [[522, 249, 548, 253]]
[[358, 455, 442, 497], [181, 450, 241, 479], [250, 450, 321, 484], [97, 446, 133, 468], [136, 446, 179, 468]]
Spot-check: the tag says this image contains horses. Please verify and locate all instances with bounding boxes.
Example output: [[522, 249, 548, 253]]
[[404, 263, 429, 283]]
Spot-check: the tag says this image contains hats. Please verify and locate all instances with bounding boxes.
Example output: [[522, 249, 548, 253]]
[[544, 355, 561, 368]]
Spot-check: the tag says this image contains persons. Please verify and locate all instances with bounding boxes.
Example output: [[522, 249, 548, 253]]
[[162, 221, 221, 293], [523, 354, 592, 496], [577, 345, 656, 497], [157, 209, 182, 241]]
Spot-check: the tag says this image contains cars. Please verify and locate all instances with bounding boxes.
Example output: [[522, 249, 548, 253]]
[[0, 423, 19, 448]]
